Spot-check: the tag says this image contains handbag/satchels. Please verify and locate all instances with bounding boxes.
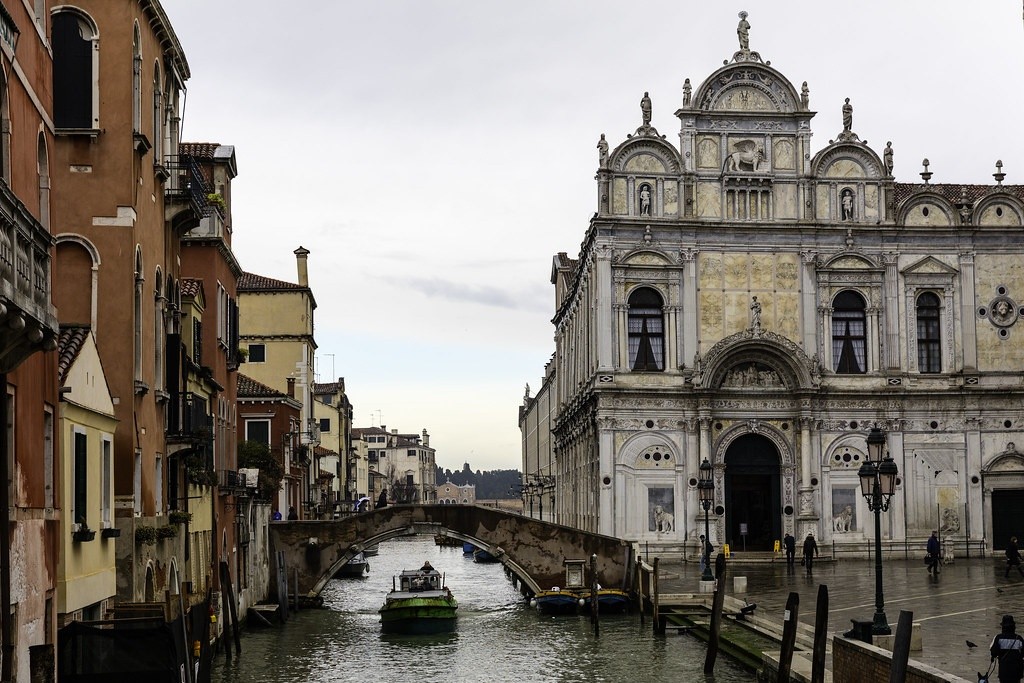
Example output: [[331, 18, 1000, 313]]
[[801, 555, 806, 566], [924, 553, 934, 564]]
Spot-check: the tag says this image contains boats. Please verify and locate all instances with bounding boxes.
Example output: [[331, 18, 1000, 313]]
[[335, 557, 370, 577], [378, 570, 459, 635]]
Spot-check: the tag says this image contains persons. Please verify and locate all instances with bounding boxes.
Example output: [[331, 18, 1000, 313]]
[[694, 350, 701, 375], [989, 615, 1024, 683], [722, 363, 780, 388], [884, 142, 893, 176], [843, 98, 853, 131], [813, 353, 819, 372], [843, 191, 852, 222], [640, 92, 652, 128], [411, 561, 434, 588], [353, 488, 387, 514], [927, 531, 942, 575], [596, 133, 609, 170], [682, 78, 692, 107], [736, 10, 750, 50], [271, 508, 282, 520], [750, 296, 761, 328], [524, 382, 530, 396], [801, 81, 809, 110], [1005, 537, 1023, 579], [288, 507, 298, 520], [699, 530, 714, 573], [640, 186, 650, 216], [784, 532, 818, 575]]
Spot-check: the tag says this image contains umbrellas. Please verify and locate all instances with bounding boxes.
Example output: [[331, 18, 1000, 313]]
[[354, 496, 371, 510]]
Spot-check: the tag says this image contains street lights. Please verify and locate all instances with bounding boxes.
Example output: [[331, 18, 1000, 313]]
[[697, 457, 716, 581], [508, 474, 556, 523], [857, 419, 901, 637]]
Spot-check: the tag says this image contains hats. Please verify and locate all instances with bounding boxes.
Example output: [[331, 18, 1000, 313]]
[[700, 535, 705, 538], [1000, 614, 1016, 626]]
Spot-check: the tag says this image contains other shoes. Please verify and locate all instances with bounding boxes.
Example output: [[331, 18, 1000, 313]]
[[932, 572, 940, 575], [926, 567, 933, 574]]
